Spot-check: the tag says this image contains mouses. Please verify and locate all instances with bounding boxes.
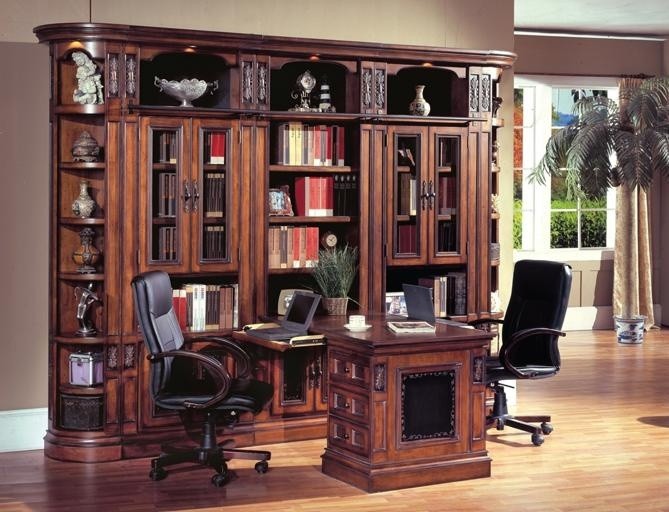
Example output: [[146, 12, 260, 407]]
[[461, 325, 475, 329]]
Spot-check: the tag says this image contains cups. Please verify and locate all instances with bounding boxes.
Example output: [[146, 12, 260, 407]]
[[349, 315, 365, 326]]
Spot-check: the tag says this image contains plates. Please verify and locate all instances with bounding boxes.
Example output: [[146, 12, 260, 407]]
[[344, 324, 372, 332]]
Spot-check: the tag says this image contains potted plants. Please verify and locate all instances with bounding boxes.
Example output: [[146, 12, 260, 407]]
[[524, 75, 669, 344]]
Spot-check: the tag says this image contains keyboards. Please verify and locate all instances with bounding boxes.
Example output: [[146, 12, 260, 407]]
[[436, 318, 468, 327]]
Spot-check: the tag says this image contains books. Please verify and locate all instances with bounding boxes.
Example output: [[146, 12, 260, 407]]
[[173, 283, 239, 332], [418, 272, 464, 318], [398, 136, 419, 253], [203, 133, 225, 259], [438, 139, 457, 252], [153, 130, 178, 260], [388, 321, 436, 334], [270, 124, 345, 165], [288, 172, 359, 216], [269, 225, 320, 268]]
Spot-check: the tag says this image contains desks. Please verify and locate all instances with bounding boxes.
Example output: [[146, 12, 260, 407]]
[[230, 312, 499, 494]]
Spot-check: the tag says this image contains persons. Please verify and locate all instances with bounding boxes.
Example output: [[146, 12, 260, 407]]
[[389, 296, 400, 315]]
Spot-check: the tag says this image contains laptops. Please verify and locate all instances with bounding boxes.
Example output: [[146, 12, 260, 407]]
[[246, 291, 323, 341]]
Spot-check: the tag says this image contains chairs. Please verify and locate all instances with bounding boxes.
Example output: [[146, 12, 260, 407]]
[[467, 259, 572, 446], [130, 269, 274, 487]]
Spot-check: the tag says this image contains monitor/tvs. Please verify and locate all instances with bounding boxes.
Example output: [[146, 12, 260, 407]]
[[402, 283, 436, 323]]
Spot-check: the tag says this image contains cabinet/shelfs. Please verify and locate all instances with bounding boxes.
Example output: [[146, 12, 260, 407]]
[[30, 21, 517, 463]]
